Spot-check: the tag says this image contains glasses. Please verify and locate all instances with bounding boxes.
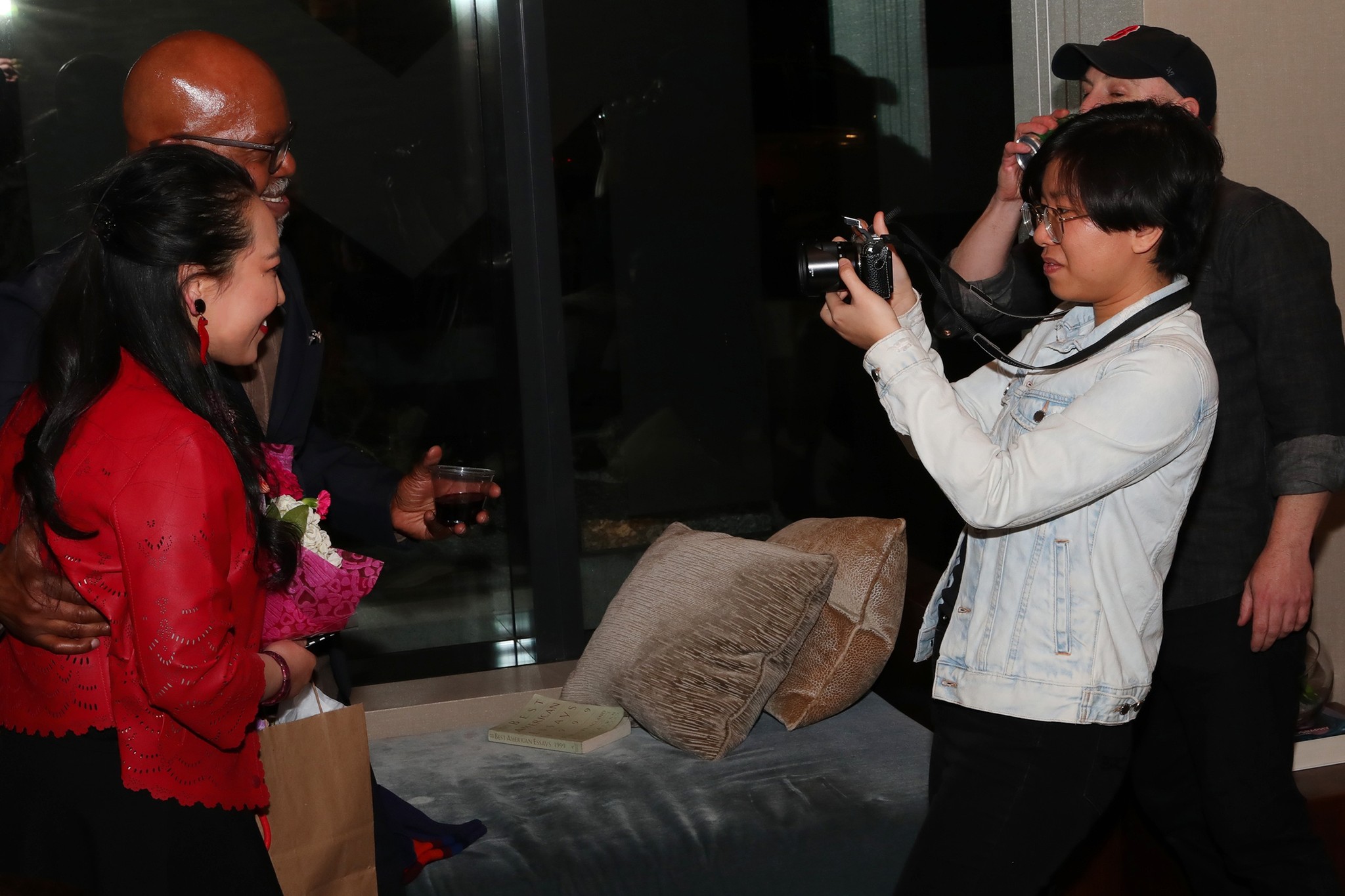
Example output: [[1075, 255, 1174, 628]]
[[147, 116, 295, 175], [1019, 201, 1091, 244]]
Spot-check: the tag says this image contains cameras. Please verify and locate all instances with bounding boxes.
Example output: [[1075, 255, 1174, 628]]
[[797, 220, 896, 303]]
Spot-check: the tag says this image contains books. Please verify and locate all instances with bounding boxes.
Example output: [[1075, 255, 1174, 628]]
[[488, 694, 632, 755]]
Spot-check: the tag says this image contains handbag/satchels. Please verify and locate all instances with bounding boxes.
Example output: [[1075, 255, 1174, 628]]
[[249, 690, 379, 895]]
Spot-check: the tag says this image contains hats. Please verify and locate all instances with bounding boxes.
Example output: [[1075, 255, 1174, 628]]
[[1051, 24, 1217, 128]]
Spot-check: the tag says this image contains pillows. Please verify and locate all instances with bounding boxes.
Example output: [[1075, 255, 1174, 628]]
[[765, 516, 908, 733], [560, 522, 839, 762]]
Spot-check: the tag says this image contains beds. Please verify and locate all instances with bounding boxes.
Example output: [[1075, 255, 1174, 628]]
[[370, 691, 934, 896]]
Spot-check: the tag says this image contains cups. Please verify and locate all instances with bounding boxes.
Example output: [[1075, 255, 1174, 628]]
[[429, 465, 495, 525]]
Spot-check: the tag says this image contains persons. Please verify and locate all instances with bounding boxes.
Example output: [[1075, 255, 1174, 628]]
[[819, 98, 1218, 896], [0, 142, 315, 896], [900, 26, 1345, 896], [0, 32, 501, 654]]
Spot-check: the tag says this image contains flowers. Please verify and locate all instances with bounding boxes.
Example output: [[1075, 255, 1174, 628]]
[[259, 466, 342, 568]]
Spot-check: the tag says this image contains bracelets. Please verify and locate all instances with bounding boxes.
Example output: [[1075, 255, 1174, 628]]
[[259, 649, 290, 710]]
